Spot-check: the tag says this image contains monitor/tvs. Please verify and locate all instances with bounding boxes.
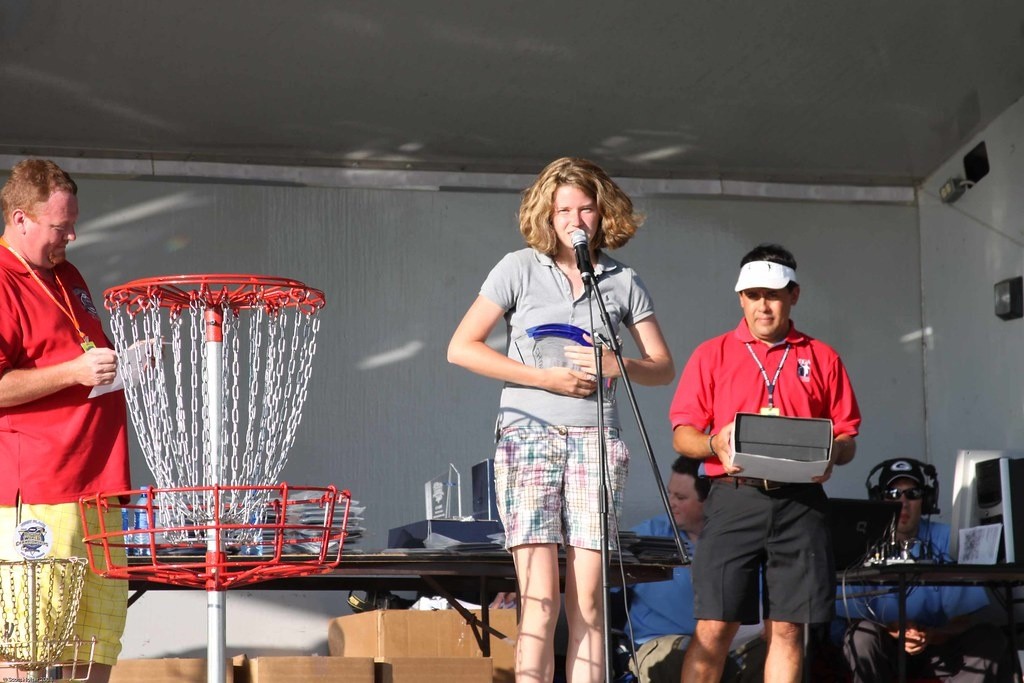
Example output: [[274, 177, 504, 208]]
[[947, 449, 1024, 562]]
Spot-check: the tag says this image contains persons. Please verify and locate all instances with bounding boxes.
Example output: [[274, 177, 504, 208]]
[[673, 245, 860, 683], [0, 155, 165, 683], [830, 458, 1010, 683], [622, 453, 770, 682], [444, 156, 678, 683]]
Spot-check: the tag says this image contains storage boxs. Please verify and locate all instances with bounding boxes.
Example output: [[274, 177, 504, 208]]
[[108, 610, 518, 683], [735, 413, 831, 462]]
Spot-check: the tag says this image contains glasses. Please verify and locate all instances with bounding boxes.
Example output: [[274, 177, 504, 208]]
[[882, 488, 922, 500]]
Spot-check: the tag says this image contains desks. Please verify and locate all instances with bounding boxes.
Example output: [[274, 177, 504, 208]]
[[804, 562, 1024, 683], [73, 555, 691, 609]]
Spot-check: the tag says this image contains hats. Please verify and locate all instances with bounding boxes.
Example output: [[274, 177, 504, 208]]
[[878, 459, 924, 486], [734, 260, 800, 293]]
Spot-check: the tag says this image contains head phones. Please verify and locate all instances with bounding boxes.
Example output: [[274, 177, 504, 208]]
[[866, 457, 940, 516]]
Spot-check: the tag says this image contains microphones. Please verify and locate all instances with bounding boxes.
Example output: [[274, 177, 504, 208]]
[[571, 230, 592, 297]]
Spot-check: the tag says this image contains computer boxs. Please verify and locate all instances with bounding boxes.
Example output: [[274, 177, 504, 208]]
[[975, 457, 1024, 564]]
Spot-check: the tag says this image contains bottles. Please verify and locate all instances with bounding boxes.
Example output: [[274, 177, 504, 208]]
[[133, 485, 156, 557], [120, 507, 130, 558], [239, 489, 266, 556]]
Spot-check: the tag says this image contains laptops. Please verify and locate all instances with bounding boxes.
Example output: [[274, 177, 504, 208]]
[[824, 497, 895, 571]]
